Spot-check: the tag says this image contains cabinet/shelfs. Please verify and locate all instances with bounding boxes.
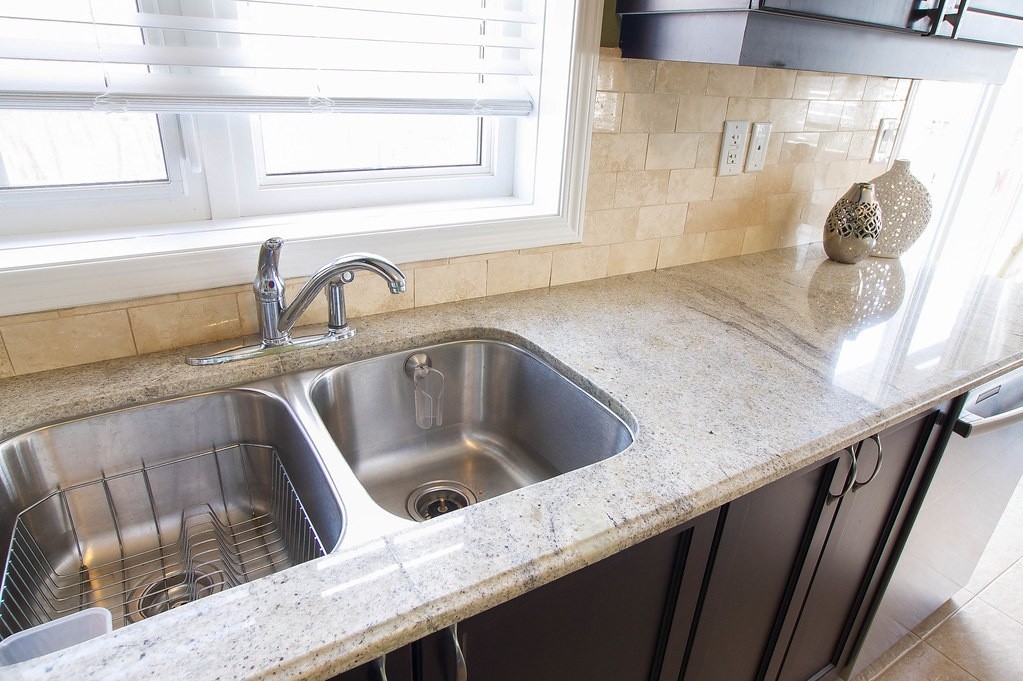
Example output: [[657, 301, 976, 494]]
[[615, 0, 1023, 86], [329, 396, 958, 681]]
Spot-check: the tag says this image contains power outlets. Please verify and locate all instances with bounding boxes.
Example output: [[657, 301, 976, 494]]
[[717, 120, 749, 177]]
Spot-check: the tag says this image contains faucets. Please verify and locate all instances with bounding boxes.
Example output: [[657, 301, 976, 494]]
[[252, 237, 406, 348]]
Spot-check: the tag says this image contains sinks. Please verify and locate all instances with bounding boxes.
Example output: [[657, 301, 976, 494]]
[[297, 325, 640, 538], [0, 364, 372, 668]]
